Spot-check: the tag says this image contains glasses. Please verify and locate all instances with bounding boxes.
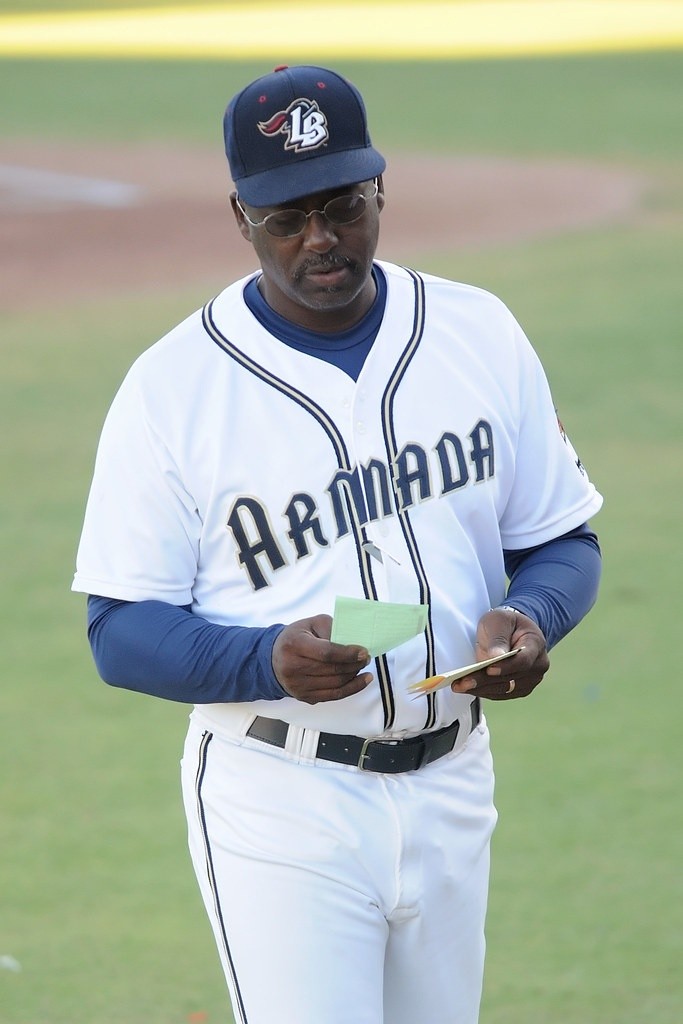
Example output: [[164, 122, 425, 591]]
[[234, 178, 380, 238]]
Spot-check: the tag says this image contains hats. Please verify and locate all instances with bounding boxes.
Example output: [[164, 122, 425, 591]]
[[223, 65, 386, 207]]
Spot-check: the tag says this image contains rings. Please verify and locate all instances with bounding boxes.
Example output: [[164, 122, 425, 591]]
[[505, 681, 515, 693]]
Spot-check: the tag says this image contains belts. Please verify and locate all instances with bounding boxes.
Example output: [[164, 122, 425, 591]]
[[245, 698, 480, 776]]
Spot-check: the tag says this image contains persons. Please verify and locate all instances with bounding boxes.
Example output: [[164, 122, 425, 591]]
[[71, 65, 603, 1023]]
